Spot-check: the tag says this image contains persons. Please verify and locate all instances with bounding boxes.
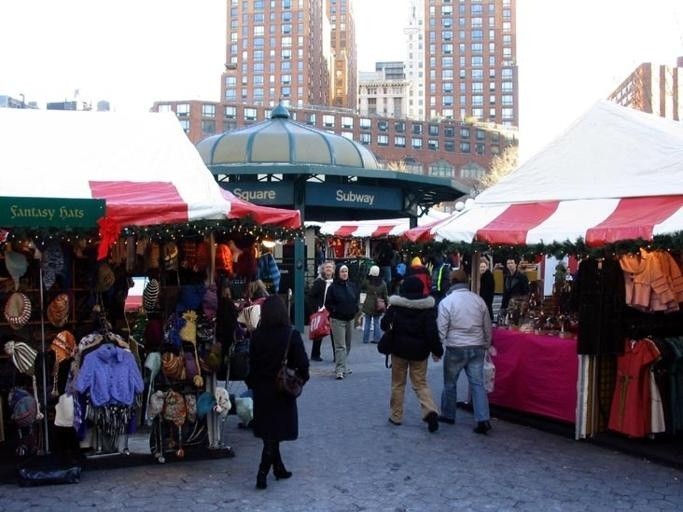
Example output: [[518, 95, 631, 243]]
[[324, 262, 359, 380], [380, 276, 444, 434], [500, 255, 530, 308], [408, 255, 432, 298], [358, 265, 389, 345], [387, 263, 407, 296], [432, 269, 493, 434], [429, 252, 451, 319], [308, 261, 336, 363], [479, 259, 495, 321], [247, 293, 311, 490]]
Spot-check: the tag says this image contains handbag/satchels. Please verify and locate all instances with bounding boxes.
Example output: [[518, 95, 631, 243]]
[[275, 325, 303, 401], [482, 348, 496, 393], [308, 306, 331, 340], [377, 328, 400, 354], [376, 298, 387, 313]]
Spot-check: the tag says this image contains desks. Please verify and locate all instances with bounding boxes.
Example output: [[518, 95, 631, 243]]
[[468, 325, 577, 442]]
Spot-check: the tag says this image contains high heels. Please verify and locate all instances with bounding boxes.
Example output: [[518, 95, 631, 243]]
[[273, 471, 292, 481], [256, 474, 268, 489]]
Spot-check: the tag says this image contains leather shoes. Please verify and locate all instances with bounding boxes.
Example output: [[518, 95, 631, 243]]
[[438, 414, 455, 424], [473, 419, 492, 434]]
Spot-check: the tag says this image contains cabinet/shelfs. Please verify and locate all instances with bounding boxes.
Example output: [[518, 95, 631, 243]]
[[0, 256, 96, 342]]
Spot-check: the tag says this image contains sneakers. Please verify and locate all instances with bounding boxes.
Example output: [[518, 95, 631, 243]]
[[343, 366, 353, 374], [389, 417, 402, 426], [336, 370, 344, 379], [427, 411, 438, 433]]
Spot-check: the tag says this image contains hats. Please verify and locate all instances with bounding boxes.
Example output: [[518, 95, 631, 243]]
[[80, 263, 231, 425], [4, 339, 39, 373], [4, 250, 28, 278], [46, 293, 72, 328], [3, 292, 33, 328], [50, 330, 77, 362], [41, 240, 66, 273], [9, 387, 41, 429]]
